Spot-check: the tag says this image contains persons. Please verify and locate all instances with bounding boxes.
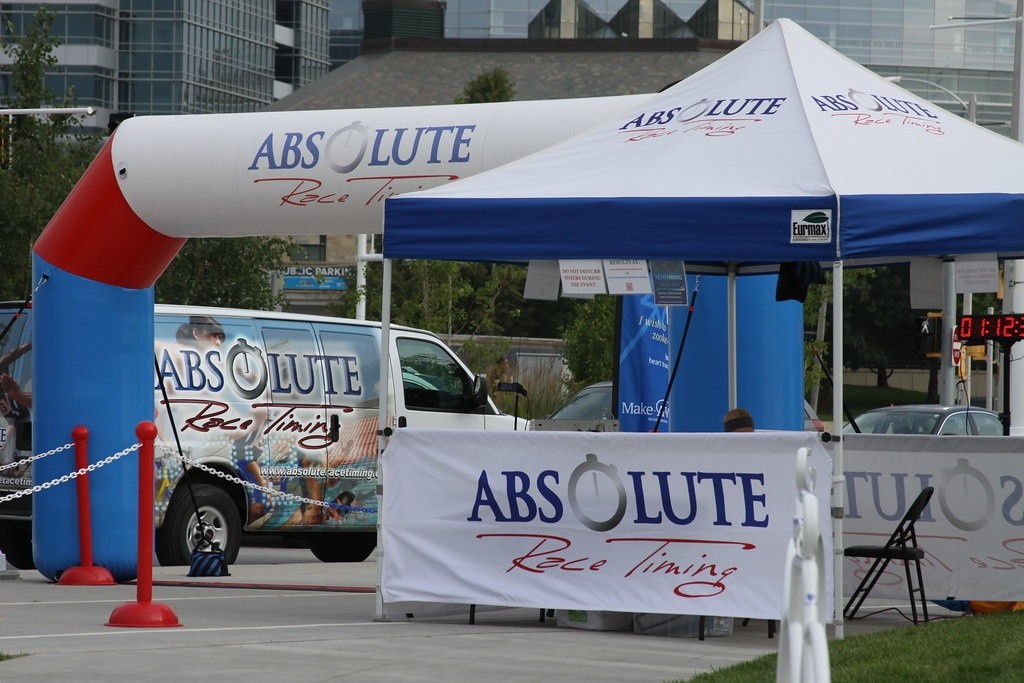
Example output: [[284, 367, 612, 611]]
[[154, 315, 324, 527], [724, 409, 754, 433], [487, 357, 514, 399], [0, 341, 32, 476]]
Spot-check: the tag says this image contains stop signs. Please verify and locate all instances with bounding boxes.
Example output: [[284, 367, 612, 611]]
[[951, 325, 962, 365]]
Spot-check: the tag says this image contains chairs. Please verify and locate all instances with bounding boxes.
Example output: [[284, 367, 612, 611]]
[[841, 486, 935, 624]]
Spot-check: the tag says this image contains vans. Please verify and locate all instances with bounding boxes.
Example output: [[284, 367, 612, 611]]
[[0, 298, 530, 568]]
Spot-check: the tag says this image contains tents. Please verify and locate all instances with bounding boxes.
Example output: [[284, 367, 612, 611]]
[[377, 16, 1024, 644]]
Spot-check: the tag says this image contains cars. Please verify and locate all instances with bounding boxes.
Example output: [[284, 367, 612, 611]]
[[546, 379, 825, 434], [841, 405, 1003, 437]]
